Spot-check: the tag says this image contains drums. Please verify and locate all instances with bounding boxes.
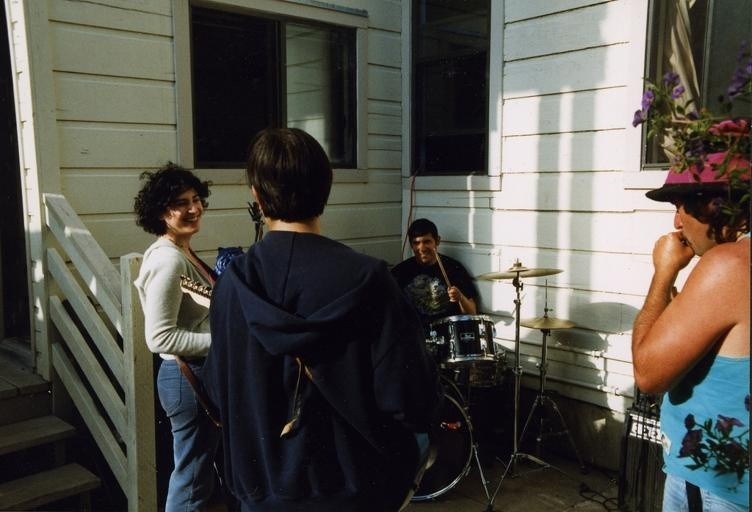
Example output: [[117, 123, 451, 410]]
[[409, 374, 473, 502], [430, 314, 500, 358], [440, 357, 498, 388]]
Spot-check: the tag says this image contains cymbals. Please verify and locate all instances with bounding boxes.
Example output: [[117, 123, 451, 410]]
[[472, 266, 562, 280], [520, 316, 576, 329]]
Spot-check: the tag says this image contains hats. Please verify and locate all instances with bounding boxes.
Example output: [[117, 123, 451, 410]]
[[644, 145, 751, 203]]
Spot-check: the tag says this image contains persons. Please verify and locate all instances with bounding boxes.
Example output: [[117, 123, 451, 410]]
[[628, 120, 752, 511], [206, 123, 438, 508], [388, 216, 478, 331], [129, 158, 221, 512]]
